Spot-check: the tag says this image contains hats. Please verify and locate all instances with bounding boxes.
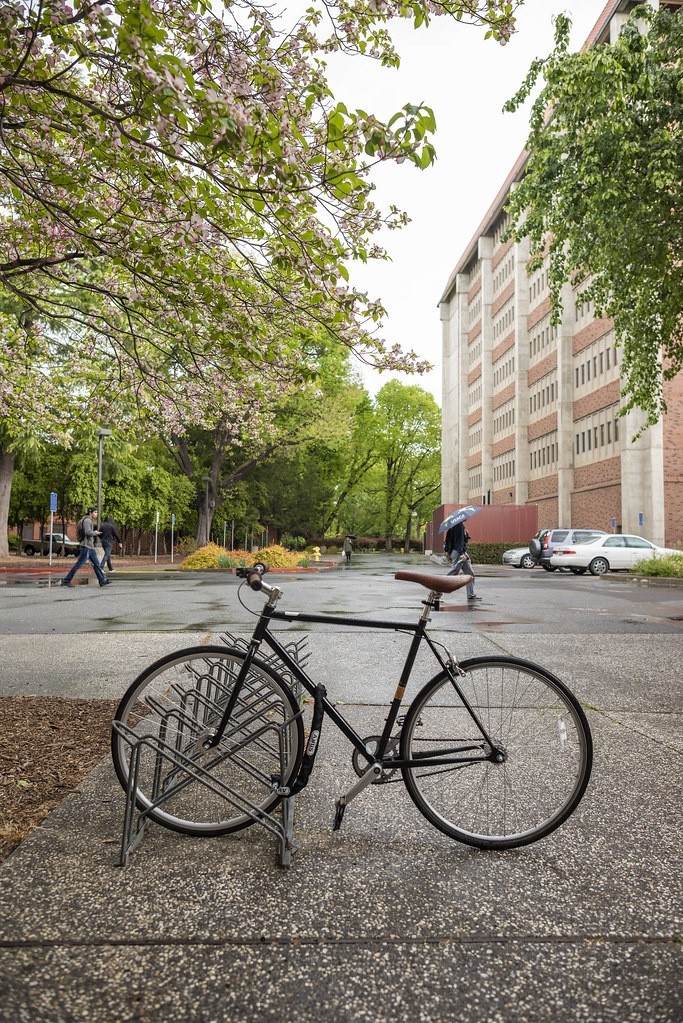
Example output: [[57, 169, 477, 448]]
[[84, 506, 96, 515]]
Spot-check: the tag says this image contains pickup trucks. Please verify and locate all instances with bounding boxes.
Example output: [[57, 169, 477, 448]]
[[21, 532, 80, 557]]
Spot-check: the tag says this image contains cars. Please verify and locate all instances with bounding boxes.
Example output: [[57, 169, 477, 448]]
[[550, 534, 683, 574], [502, 546, 539, 569]]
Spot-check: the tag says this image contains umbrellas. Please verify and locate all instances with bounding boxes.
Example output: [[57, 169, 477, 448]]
[[345, 535, 357, 541], [439, 505, 482, 534]]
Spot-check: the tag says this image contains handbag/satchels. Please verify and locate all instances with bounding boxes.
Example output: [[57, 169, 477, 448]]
[[342, 550, 345, 556]]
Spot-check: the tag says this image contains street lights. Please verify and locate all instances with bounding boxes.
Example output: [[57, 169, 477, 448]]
[[94, 429, 112, 546], [412, 510, 417, 539], [321, 506, 327, 546], [202, 476, 212, 544]]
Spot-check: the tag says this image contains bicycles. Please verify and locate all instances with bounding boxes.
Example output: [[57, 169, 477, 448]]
[[112, 560, 593, 850]]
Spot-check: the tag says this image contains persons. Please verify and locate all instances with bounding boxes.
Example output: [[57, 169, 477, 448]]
[[99, 518, 122, 573], [344, 537, 353, 562], [62, 507, 112, 587], [434, 519, 482, 601]]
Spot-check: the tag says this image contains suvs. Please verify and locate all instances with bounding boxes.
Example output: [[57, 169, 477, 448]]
[[529, 528, 621, 574]]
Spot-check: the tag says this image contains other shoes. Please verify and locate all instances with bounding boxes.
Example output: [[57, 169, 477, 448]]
[[100, 580, 112, 587], [429, 596, 444, 603], [467, 595, 482, 601], [109, 569, 115, 573], [61, 582, 75, 587], [102, 570, 106, 573]]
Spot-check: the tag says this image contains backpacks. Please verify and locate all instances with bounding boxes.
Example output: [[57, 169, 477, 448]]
[[77, 517, 92, 541], [445, 529, 455, 554]]
